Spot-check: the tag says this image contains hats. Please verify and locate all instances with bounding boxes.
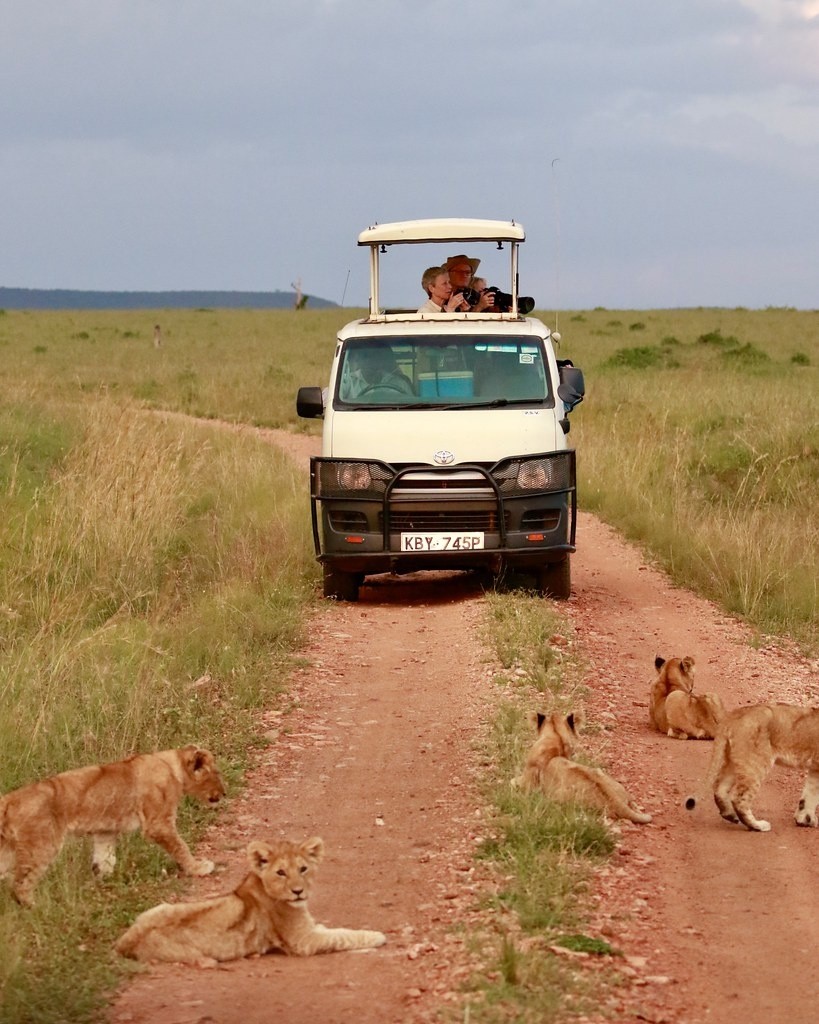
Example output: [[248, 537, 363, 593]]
[[440, 255, 482, 275]]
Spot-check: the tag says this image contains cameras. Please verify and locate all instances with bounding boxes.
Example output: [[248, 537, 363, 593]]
[[453, 287, 481, 306], [482, 286, 535, 314]]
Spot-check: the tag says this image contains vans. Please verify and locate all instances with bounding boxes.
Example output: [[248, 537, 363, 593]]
[[295, 215, 586, 606]]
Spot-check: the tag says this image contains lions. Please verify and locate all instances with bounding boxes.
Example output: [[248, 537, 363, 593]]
[[518, 710, 653, 824], [0, 744, 227, 909], [648, 655, 727, 740], [684, 702, 819, 832], [114, 836, 388, 969]]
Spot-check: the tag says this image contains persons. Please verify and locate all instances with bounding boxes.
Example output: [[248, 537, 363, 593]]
[[320, 341, 414, 411], [413, 254, 520, 397]]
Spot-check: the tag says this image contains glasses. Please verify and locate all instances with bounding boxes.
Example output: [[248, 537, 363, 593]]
[[449, 269, 471, 275]]
